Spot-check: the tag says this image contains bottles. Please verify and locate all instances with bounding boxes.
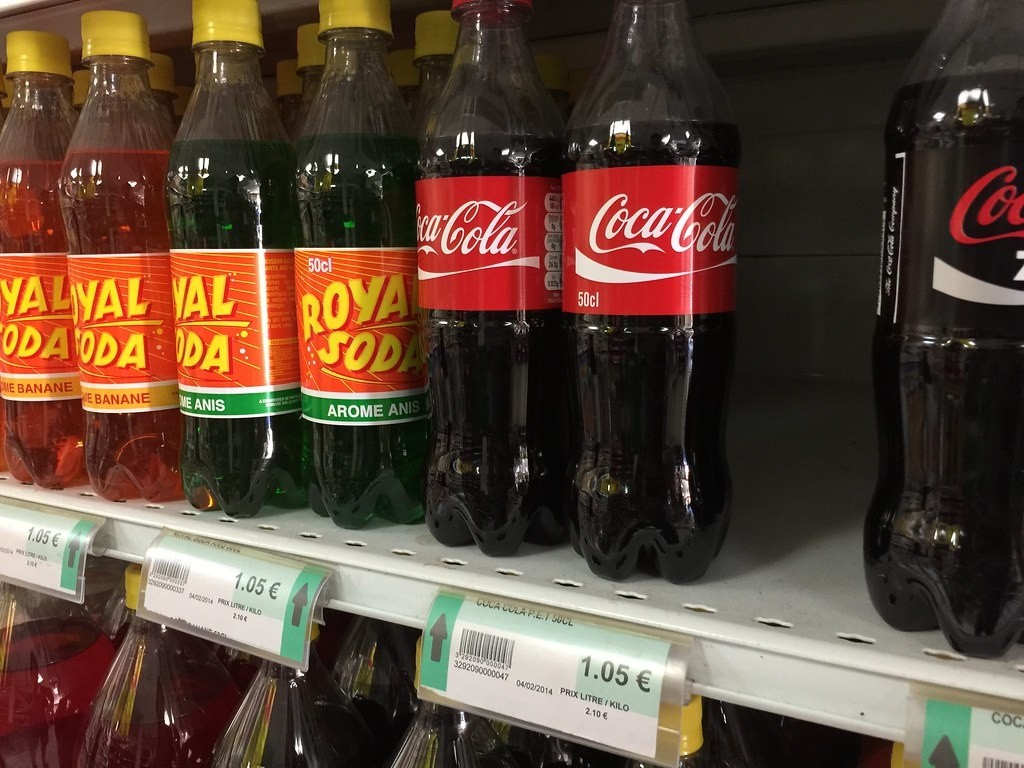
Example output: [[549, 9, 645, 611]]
[[330, 611, 423, 741], [862, 2, 1023, 658], [0, 1, 741, 582], [0, 582, 116, 768], [76, 562, 243, 768], [205, 621, 376, 768], [376, 633, 519, 768]]
